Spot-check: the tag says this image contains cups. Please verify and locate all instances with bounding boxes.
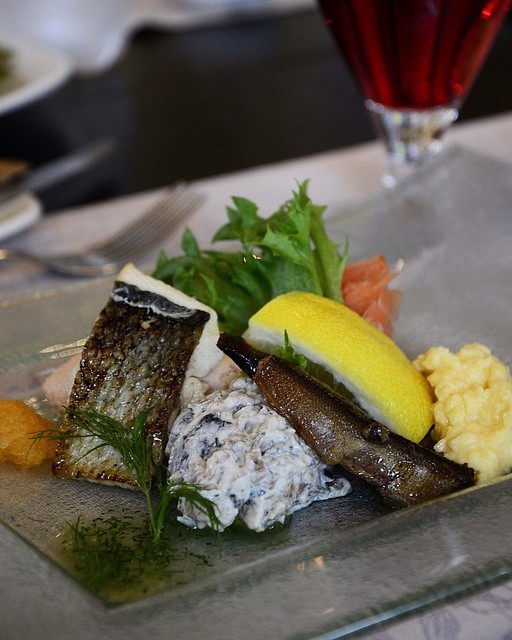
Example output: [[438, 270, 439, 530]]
[[315, 0, 512, 193]]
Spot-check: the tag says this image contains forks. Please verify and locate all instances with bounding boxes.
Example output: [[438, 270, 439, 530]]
[[0, 179, 208, 281]]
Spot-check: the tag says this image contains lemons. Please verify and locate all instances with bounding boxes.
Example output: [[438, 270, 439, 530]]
[[240, 289, 434, 443]]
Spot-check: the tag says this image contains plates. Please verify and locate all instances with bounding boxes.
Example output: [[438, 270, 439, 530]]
[[0, 37, 76, 118], [0, 144, 512, 640]]
